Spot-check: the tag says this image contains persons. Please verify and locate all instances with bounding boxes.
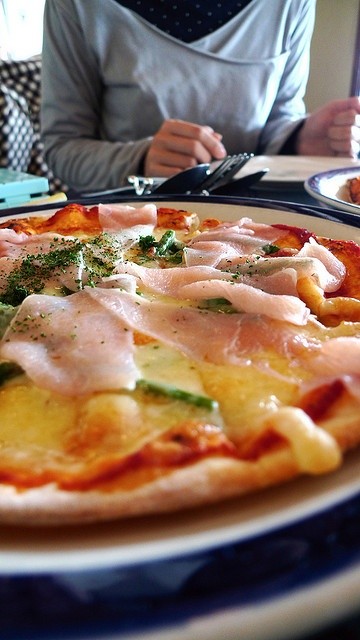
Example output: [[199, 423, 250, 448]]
[[39, 0, 360, 194]]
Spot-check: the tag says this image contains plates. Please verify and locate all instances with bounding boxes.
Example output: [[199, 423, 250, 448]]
[[0, 194, 360, 640], [303, 165, 360, 215], [206, 154, 360, 183]]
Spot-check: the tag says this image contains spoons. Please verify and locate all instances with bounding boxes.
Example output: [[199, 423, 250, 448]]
[[150, 163, 211, 195], [208, 167, 271, 196]]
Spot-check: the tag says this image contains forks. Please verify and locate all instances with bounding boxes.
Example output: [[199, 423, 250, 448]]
[[191, 152, 255, 194]]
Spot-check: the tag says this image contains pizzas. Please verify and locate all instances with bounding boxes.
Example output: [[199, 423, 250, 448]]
[[0, 203, 360, 529]]
